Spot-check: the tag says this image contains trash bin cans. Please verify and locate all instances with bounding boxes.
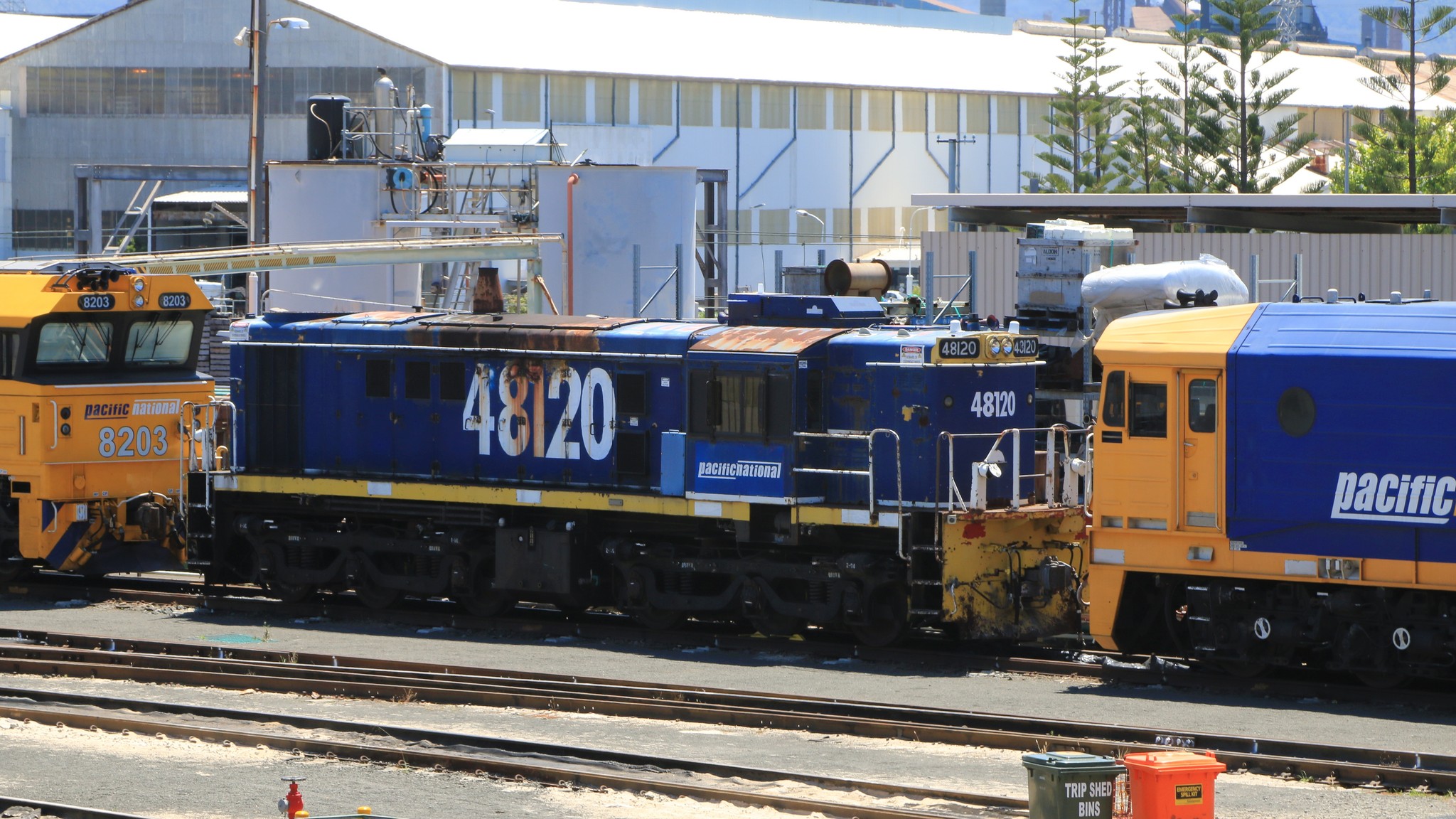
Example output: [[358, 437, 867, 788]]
[[1123, 752, 1225, 819], [1020, 752, 1126, 819]]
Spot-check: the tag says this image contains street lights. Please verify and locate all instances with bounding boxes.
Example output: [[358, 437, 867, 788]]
[[1343, 104, 1355, 194], [905, 206, 949, 297], [795, 209, 825, 244], [485, 109, 495, 129], [245, 19, 310, 317]]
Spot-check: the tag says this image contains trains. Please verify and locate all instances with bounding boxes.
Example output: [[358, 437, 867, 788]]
[[0, 260, 1456, 688]]
